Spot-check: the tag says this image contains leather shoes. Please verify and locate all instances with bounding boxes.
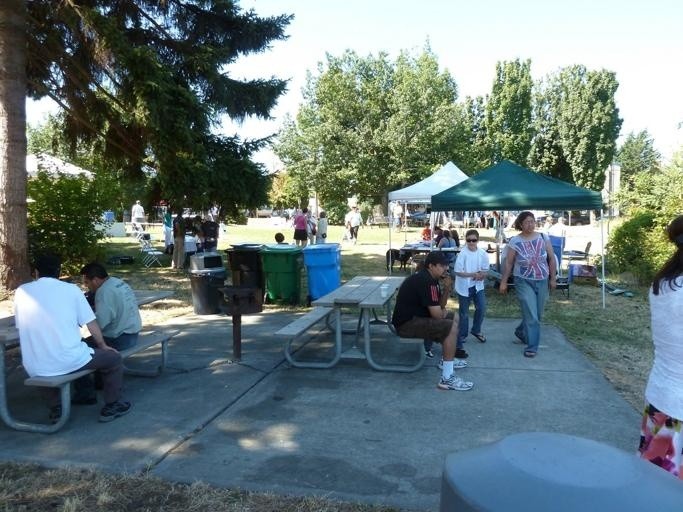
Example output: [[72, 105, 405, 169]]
[[71, 391, 97, 405]]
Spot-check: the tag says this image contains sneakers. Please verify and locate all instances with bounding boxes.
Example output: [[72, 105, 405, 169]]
[[437, 373, 473, 391], [425, 350, 434, 358], [437, 348, 469, 370], [49, 405, 61, 423], [97, 400, 131, 422]]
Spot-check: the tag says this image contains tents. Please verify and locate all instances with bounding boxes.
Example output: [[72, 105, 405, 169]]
[[388, 162, 470, 248]]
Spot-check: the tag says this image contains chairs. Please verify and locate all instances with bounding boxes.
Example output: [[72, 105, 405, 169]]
[[125, 221, 173, 269], [548, 235, 598, 300], [365, 215, 478, 230]]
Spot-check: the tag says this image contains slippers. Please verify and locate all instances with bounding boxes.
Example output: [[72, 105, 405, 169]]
[[470, 331, 486, 343], [524, 351, 535, 357], [514, 331, 527, 344]]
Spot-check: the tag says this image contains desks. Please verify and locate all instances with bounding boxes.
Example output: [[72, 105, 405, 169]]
[[0, 289, 177, 432], [398, 241, 502, 275], [309, 275, 407, 372]]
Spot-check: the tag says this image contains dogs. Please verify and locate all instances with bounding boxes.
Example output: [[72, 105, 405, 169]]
[[386, 248, 419, 272]]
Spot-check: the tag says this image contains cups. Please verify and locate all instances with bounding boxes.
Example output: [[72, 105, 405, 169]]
[[379, 283, 390, 299]]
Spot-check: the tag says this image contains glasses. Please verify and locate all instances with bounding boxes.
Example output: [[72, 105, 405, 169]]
[[466, 239, 476, 242]]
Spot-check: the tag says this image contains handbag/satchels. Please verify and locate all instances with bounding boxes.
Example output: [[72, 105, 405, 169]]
[[547, 254, 559, 282]]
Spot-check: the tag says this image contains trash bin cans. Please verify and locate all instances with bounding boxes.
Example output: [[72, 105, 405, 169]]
[[187, 243, 341, 315]]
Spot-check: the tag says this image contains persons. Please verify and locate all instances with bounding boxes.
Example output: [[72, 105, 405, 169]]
[[391, 252, 473, 390], [13, 250, 134, 423], [72, 261, 141, 404], [275, 207, 328, 249], [638, 215, 681, 473], [423, 280, 469, 358], [131, 201, 221, 272], [344, 204, 364, 240], [394, 203, 403, 228], [366, 214, 372, 224], [500, 211, 556, 358], [452, 229, 488, 343]]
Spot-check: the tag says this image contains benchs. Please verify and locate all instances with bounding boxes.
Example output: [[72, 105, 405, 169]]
[[397, 336, 426, 372], [20, 326, 181, 433], [272, 307, 335, 369]]
